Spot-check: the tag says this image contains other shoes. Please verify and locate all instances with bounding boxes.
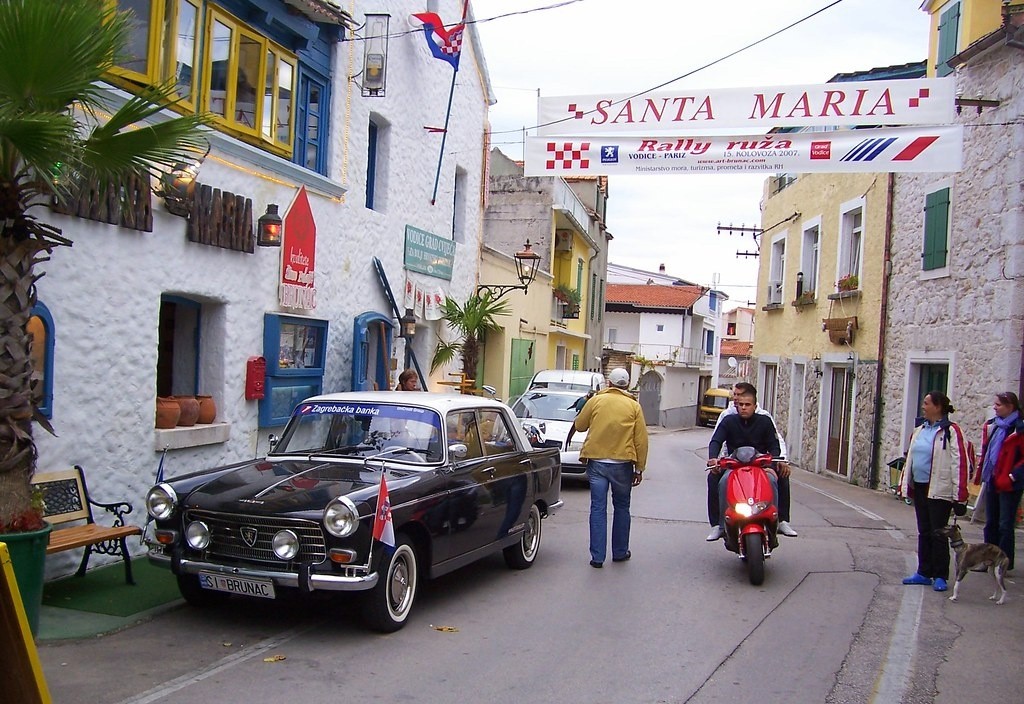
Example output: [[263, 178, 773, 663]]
[[934, 578, 947, 591], [706, 525, 725, 541], [903, 572, 932, 585], [971, 562, 988, 572], [612, 551, 631, 562], [590, 559, 602, 568], [776, 520, 798, 537]]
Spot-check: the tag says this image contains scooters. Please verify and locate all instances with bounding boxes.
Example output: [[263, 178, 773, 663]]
[[481, 384, 546, 448], [704, 446, 789, 586]]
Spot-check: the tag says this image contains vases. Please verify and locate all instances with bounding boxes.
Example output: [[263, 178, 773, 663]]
[[802, 290, 815, 299], [838, 283, 859, 291], [156, 392, 215, 428]]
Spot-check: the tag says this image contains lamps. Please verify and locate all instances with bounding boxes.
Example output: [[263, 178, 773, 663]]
[[391, 311, 419, 336], [344, 11, 392, 98], [478, 240, 541, 294], [166, 130, 211, 217], [253, 203, 284, 248]]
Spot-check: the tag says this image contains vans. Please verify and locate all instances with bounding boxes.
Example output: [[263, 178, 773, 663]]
[[525, 368, 606, 413], [699, 387, 733, 427]]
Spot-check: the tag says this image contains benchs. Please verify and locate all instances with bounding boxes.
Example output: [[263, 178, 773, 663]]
[[32, 465, 142, 586]]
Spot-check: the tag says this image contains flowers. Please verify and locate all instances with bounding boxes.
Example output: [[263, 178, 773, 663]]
[[836, 273, 861, 286]]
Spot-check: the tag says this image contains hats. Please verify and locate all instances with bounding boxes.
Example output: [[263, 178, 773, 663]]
[[609, 368, 629, 387]]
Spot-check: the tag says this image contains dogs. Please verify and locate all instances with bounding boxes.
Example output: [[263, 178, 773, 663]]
[[930, 524, 1009, 605]]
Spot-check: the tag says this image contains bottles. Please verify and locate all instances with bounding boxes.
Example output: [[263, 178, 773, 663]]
[[196, 395, 216, 424], [174, 394, 200, 426], [156, 397, 181, 429], [366, 21, 384, 89]]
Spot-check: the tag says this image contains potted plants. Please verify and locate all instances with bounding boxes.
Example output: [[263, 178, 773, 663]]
[[0, 0, 215, 636], [553, 285, 581, 308]]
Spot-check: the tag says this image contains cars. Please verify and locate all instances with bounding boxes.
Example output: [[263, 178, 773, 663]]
[[510, 384, 592, 490], [139, 391, 564, 634]]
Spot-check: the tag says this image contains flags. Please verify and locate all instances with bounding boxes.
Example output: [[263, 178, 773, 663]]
[[411, 13, 467, 71], [374, 475, 396, 558]]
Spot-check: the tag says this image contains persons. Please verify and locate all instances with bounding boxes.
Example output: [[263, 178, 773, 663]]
[[973, 391, 1024, 574], [574, 370, 647, 568], [897, 390, 969, 591], [395, 369, 422, 391], [706, 384, 799, 541]]
